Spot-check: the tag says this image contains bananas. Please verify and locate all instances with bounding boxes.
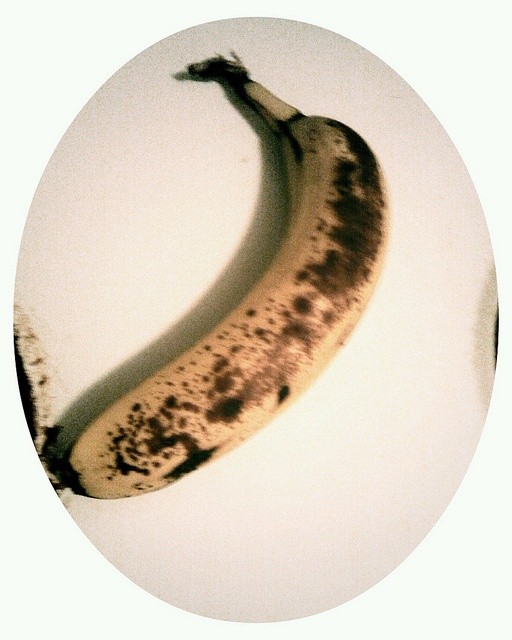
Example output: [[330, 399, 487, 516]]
[[65, 49, 391, 501]]
